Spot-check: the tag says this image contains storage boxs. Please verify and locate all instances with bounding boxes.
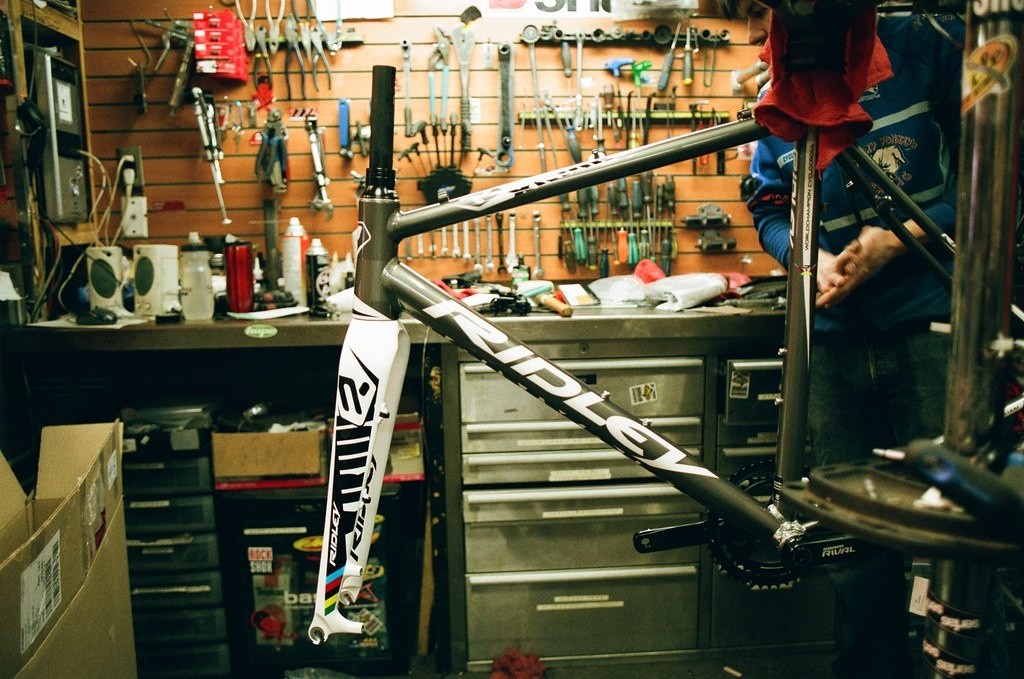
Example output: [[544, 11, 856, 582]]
[[211, 427, 328, 490], [242, 525, 389, 668], [329, 414, 425, 483], [0, 418, 137, 679]]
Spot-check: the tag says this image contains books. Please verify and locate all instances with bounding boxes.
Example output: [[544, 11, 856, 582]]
[[145, 397, 224, 429], [558, 283, 595, 307]]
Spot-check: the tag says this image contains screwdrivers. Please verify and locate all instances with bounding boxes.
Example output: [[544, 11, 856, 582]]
[[683, 20, 693, 86], [560, 41, 572, 102], [558, 169, 676, 279], [658, 21, 681, 91]]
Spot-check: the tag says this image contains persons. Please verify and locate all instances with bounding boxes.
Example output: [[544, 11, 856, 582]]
[[718, 0, 1010, 679]]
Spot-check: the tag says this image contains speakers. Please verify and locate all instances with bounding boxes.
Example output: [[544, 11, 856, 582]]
[[86, 245, 134, 319], [131, 243, 180, 318]]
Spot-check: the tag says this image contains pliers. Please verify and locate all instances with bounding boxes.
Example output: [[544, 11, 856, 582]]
[[291, 0, 312, 63], [235, 0, 258, 51], [265, 0, 285, 56], [253, 24, 273, 91], [310, 26, 332, 92], [284, 13, 307, 101]]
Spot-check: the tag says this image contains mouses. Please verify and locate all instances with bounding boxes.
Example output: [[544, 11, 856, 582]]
[[78, 308, 115, 325]]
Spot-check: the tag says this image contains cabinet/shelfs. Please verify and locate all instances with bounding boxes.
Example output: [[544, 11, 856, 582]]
[[123, 429, 233, 679], [436, 339, 843, 674]]
